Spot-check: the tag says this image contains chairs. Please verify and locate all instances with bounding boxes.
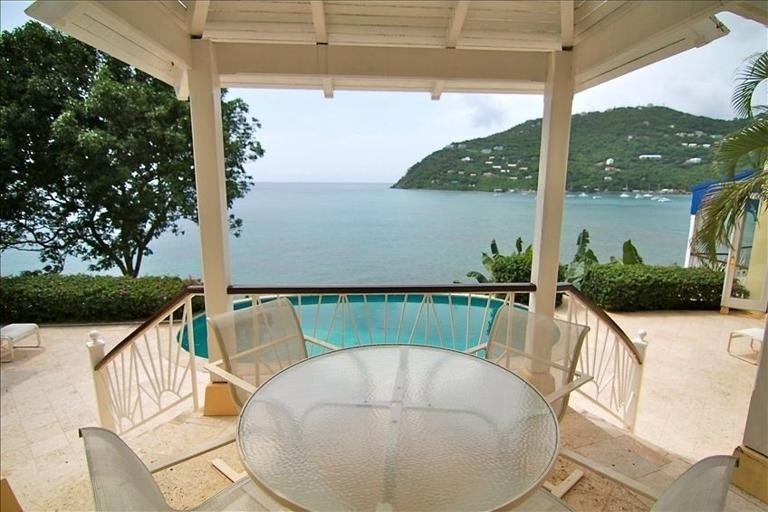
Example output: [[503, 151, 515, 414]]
[[503, 453, 737, 510], [206, 296, 343, 510], [79, 426, 289, 509], [460, 302, 587, 502]]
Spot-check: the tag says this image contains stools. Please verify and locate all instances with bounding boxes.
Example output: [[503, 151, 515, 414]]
[[1, 322, 43, 365], [728, 327, 765, 367]]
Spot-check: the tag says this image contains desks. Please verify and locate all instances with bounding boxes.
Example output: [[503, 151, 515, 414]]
[[232, 342, 561, 510]]
